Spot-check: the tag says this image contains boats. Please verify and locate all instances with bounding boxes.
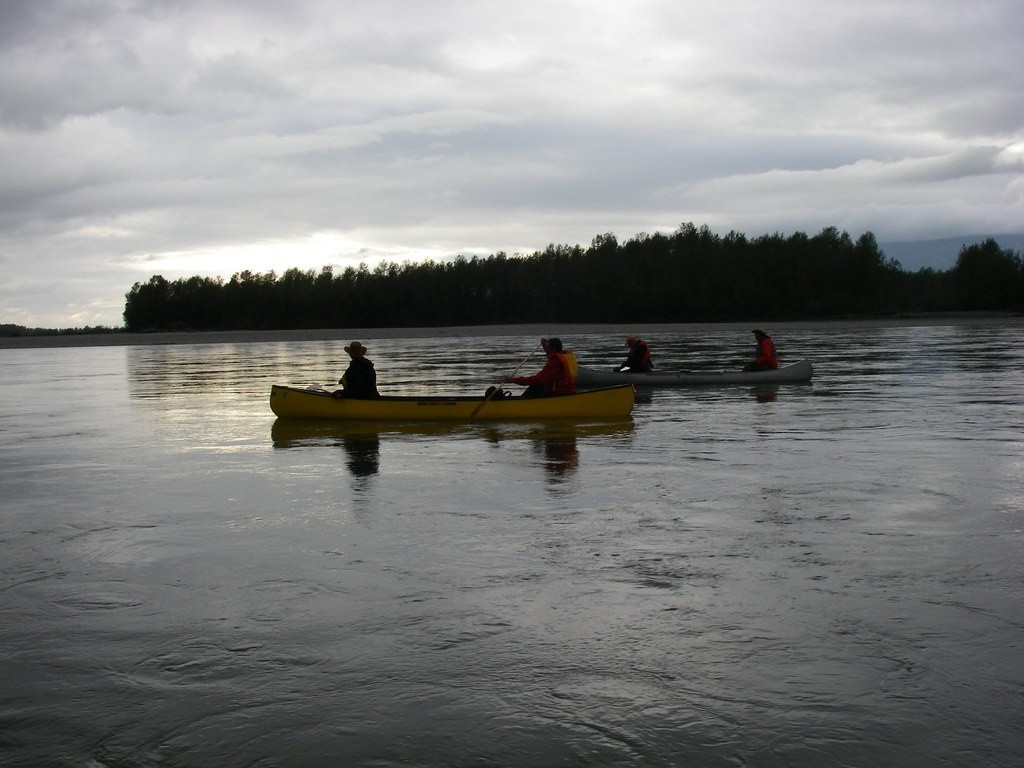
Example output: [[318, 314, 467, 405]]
[[577, 358, 815, 385], [267, 381, 639, 422]]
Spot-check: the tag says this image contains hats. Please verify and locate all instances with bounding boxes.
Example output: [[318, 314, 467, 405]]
[[344, 342, 367, 358], [626, 336, 636, 345], [752, 328, 769, 337]]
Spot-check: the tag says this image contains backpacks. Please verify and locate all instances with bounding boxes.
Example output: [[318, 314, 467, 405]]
[[485, 386, 512, 400]]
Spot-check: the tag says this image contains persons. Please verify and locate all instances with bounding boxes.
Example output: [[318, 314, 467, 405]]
[[742, 330, 777, 371], [504, 337, 578, 399], [615, 335, 653, 374], [334, 341, 377, 398]]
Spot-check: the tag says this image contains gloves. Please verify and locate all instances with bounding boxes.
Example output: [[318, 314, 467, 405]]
[[613, 367, 620, 373]]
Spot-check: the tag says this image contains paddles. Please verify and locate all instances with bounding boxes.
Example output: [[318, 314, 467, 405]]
[[469, 341, 544, 421]]
